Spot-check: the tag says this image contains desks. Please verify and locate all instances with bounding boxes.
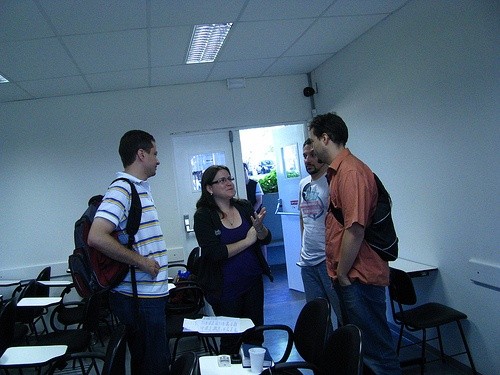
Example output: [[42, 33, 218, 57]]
[[0, 280, 20, 287], [17, 297, 63, 337], [198, 356, 275, 375], [37, 280, 73, 287], [386, 258, 439, 279], [0, 345, 68, 375]]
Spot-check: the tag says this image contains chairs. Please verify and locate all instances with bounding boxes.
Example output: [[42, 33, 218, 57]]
[[0, 266, 129, 375], [388, 267, 477, 375], [162, 247, 363, 375]]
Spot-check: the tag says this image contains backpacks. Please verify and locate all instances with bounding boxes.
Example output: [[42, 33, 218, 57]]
[[69, 178, 142, 315], [327, 173, 398, 261]]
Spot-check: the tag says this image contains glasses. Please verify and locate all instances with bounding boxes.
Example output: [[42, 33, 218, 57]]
[[302, 183, 311, 202], [212, 176, 234, 184]]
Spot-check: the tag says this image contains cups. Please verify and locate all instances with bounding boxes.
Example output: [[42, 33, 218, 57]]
[[248, 347, 266, 371]]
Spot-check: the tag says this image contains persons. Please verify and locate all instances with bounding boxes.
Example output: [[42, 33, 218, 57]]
[[298, 138, 342, 340], [193, 165, 272, 355], [87, 130, 171, 375], [308, 112, 403, 375], [243, 163, 267, 262]]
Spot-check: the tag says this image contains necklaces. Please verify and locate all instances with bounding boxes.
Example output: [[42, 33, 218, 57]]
[[223, 205, 234, 226]]
